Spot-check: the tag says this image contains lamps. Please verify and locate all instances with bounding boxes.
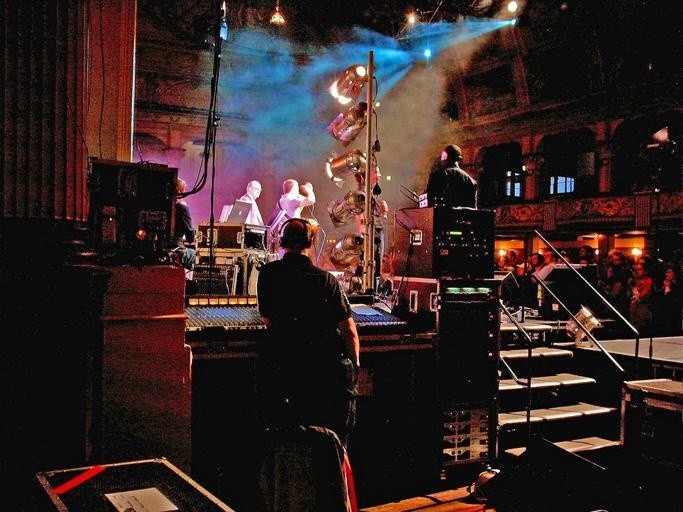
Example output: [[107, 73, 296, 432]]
[[323, 49, 379, 294]]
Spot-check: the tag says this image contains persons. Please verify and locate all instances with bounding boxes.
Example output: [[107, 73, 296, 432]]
[[258, 218, 360, 455], [495, 243, 682, 331], [423, 143, 479, 208], [275, 179, 317, 257], [239, 180, 263, 226], [174, 177, 194, 244]]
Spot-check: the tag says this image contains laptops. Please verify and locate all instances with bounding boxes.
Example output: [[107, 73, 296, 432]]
[[223, 199, 252, 223]]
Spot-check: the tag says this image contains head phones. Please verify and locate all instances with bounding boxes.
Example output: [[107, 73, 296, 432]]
[[277, 218, 316, 248]]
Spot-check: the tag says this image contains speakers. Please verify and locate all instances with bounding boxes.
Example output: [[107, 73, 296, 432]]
[[394, 207, 496, 279]]
